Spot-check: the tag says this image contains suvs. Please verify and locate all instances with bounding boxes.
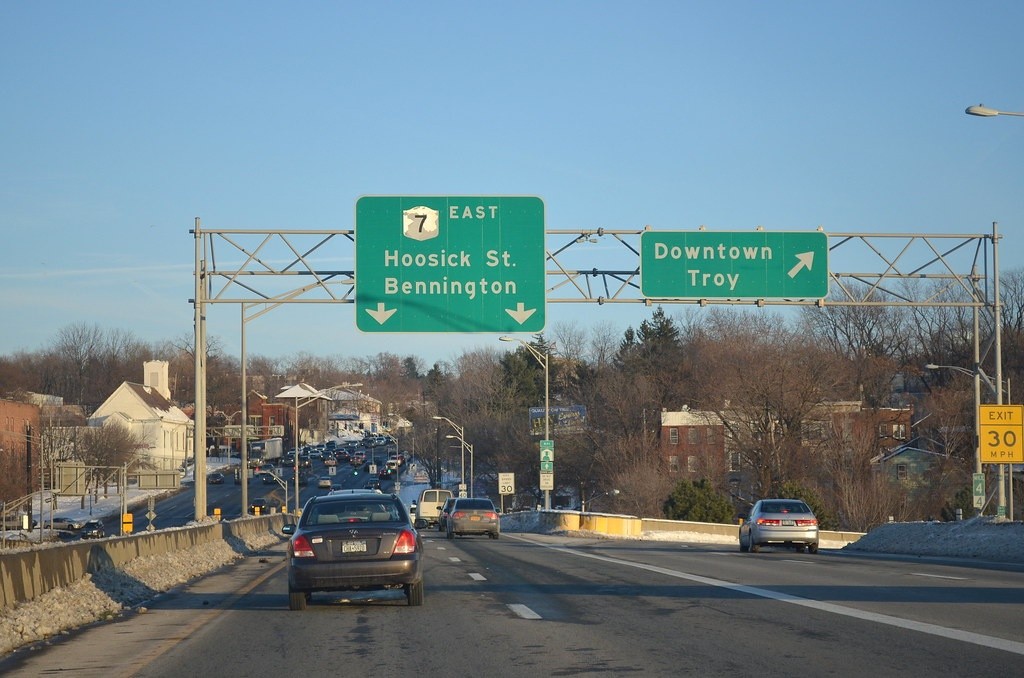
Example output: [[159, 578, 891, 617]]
[[79, 519, 105, 540]]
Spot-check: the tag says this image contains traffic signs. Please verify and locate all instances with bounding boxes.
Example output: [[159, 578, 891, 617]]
[[353, 193, 547, 335], [639, 230, 830, 302]]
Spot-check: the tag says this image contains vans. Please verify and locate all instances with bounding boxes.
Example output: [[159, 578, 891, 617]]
[[411, 489, 456, 524]]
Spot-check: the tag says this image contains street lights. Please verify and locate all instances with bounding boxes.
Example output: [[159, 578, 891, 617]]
[[433, 416, 464, 484], [373, 432, 398, 484], [444, 436, 473, 498], [213, 409, 242, 467]]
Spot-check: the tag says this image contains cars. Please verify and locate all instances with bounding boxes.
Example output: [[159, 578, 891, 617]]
[[362, 482, 382, 491], [230, 429, 430, 484], [251, 498, 268, 513], [318, 476, 331, 489], [0, 515, 37, 529], [44, 516, 81, 531], [436, 498, 460, 532], [207, 472, 224, 485], [281, 490, 430, 610], [738, 499, 819, 554], [443, 497, 502, 539], [329, 484, 343, 492], [293, 470, 308, 487]]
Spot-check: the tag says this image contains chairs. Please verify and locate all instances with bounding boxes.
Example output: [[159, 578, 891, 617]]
[[371, 512, 391, 521], [318, 514, 339, 523]]
